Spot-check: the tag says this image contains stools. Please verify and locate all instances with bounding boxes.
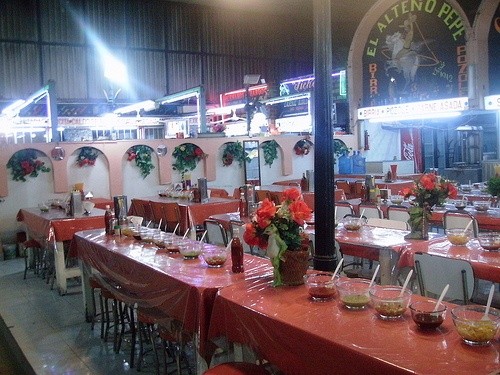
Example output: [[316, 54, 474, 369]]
[[20, 242, 274, 375]]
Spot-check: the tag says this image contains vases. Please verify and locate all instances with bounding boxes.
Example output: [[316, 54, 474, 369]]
[[419, 207, 433, 240], [279, 250, 310, 285]]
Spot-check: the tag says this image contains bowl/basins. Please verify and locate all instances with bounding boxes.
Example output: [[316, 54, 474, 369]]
[[303, 212, 315, 226], [408, 299, 449, 332], [369, 285, 413, 322], [476, 232, 500, 252], [445, 227, 475, 247], [437, 182, 492, 214], [122, 225, 231, 268], [333, 277, 376, 311], [38, 198, 70, 215], [158, 189, 194, 201], [388, 194, 404, 205], [303, 273, 341, 303], [451, 304, 500, 347], [342, 218, 364, 232]]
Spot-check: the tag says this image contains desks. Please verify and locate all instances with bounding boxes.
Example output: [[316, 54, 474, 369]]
[[17, 203, 115, 293], [74, 224, 271, 375], [208, 271, 500, 375], [395, 233, 500, 282], [210, 206, 440, 284], [253, 167, 500, 230], [138, 188, 241, 242]]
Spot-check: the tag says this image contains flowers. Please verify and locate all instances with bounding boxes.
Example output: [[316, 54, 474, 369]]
[[7, 150, 51, 182], [75, 147, 98, 167], [222, 141, 251, 168], [126, 145, 154, 179], [399, 172, 457, 206], [170, 144, 206, 175], [294, 139, 314, 155], [243, 189, 313, 250]]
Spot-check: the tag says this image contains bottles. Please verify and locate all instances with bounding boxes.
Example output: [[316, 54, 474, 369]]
[[301, 172, 309, 192], [239, 193, 248, 217], [338, 150, 393, 204], [105, 205, 140, 236], [194, 184, 200, 203], [231, 229, 244, 273]]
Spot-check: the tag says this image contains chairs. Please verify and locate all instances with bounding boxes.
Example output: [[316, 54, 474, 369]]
[[129, 179, 480, 306]]
